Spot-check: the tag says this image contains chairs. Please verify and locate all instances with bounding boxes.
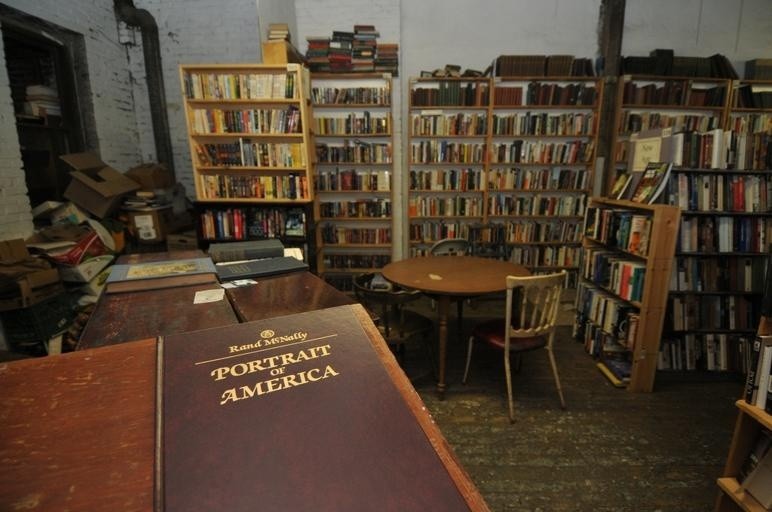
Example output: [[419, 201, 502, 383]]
[[429, 238, 471, 309], [461, 269, 566, 423], [352, 271, 439, 383]]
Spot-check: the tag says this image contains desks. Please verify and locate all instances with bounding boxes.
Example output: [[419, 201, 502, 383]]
[[0, 304, 491, 512], [220, 270, 358, 323], [382, 256, 533, 400], [76, 249, 240, 351]]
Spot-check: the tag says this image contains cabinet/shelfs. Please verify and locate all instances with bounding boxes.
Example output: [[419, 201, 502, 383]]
[[310, 72, 396, 295], [571, 197, 682, 395], [406, 75, 604, 303], [606, 74, 732, 198], [714, 317, 772, 512], [178, 63, 323, 283], [613, 160, 772, 381], [725, 80, 772, 131]]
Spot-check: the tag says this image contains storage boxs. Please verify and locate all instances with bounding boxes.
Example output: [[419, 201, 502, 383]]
[[1, 268, 76, 345], [126, 163, 169, 191], [128, 207, 174, 243], [59, 152, 141, 219]]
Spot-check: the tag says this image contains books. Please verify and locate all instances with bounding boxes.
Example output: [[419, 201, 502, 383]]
[[493, 112, 597, 137], [533, 269, 578, 289], [23, 83, 62, 117], [409, 193, 482, 217], [313, 111, 391, 134], [315, 140, 392, 165], [623, 172, 644, 200], [619, 111, 720, 135], [491, 245, 583, 267], [765, 361, 772, 416], [745, 58, 772, 79], [596, 362, 631, 388], [744, 335, 772, 405], [410, 168, 486, 191], [526, 82, 601, 106], [620, 54, 738, 79], [674, 213, 772, 251], [489, 167, 592, 190], [312, 87, 390, 104], [623, 79, 726, 107], [736, 430, 772, 484], [106, 256, 218, 293], [584, 208, 652, 257], [283, 208, 306, 236], [200, 207, 284, 239], [188, 108, 302, 134], [495, 86, 522, 106], [409, 223, 484, 243], [328, 31, 354, 74], [756, 345, 772, 410], [724, 114, 772, 134], [491, 140, 593, 163], [216, 255, 309, 282], [488, 193, 585, 215], [209, 239, 284, 264], [197, 173, 308, 200], [667, 171, 772, 212], [630, 161, 673, 206], [742, 448, 772, 511], [186, 71, 300, 100], [609, 173, 634, 201], [318, 198, 393, 220], [375, 42, 399, 77], [409, 114, 487, 137], [410, 246, 430, 258], [352, 25, 380, 73], [320, 223, 391, 243], [669, 254, 772, 291], [733, 84, 772, 109], [410, 81, 489, 106], [649, 49, 675, 58], [420, 109, 442, 115], [313, 170, 392, 192], [572, 314, 625, 358], [409, 139, 486, 165], [574, 281, 640, 351], [194, 137, 306, 169], [617, 135, 772, 169], [582, 241, 647, 301], [304, 35, 330, 73], [668, 292, 772, 332], [488, 221, 584, 244], [325, 251, 391, 268], [497, 55, 594, 77], [324, 273, 357, 292], [155, 307, 471, 512], [267, 23, 291, 43], [658, 334, 751, 374], [601, 355, 633, 382]]
